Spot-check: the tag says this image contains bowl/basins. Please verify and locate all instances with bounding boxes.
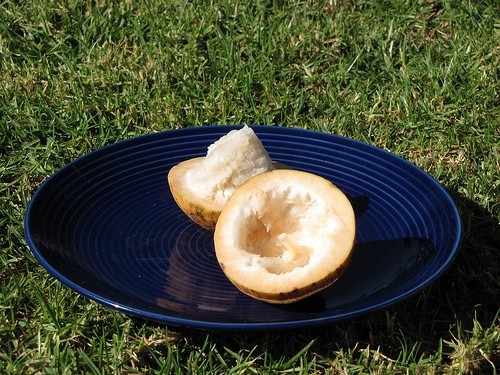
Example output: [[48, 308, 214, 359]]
[[24, 124, 462, 334]]
